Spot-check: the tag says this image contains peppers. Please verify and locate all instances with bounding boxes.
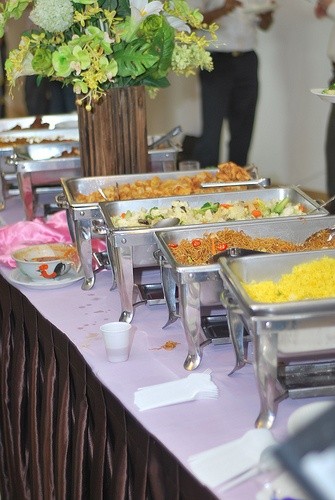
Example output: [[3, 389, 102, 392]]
[[218, 243, 227, 251], [192, 240, 202, 247], [168, 243, 178, 248]]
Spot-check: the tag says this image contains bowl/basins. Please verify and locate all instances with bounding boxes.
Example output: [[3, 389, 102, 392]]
[[11, 243, 76, 281]]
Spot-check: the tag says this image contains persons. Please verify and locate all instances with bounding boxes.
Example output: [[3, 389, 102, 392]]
[[188, 1, 274, 168], [316, 1, 335, 212]]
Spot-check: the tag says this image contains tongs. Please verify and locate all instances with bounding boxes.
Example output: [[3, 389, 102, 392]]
[[201, 176, 272, 188], [148, 125, 181, 150], [97, 182, 122, 202]]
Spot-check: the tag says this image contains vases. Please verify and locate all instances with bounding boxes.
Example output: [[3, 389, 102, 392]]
[[77, 87, 150, 176]]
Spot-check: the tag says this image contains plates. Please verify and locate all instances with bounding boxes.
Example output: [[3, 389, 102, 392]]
[[9, 268, 86, 291]]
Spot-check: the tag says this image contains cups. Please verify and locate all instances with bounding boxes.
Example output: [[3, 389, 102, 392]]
[[100, 322, 132, 363]]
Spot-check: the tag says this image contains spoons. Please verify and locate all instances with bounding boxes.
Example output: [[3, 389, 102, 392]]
[[308, 194, 335, 213], [97, 217, 180, 230]]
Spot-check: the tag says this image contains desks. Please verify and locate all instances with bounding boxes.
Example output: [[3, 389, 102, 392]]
[[0, 214, 333, 500]]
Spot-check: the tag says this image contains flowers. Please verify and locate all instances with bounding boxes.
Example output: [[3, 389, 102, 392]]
[[0, 0, 219, 110]]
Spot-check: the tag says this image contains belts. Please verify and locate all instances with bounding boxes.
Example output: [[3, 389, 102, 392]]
[[210, 51, 257, 58]]
